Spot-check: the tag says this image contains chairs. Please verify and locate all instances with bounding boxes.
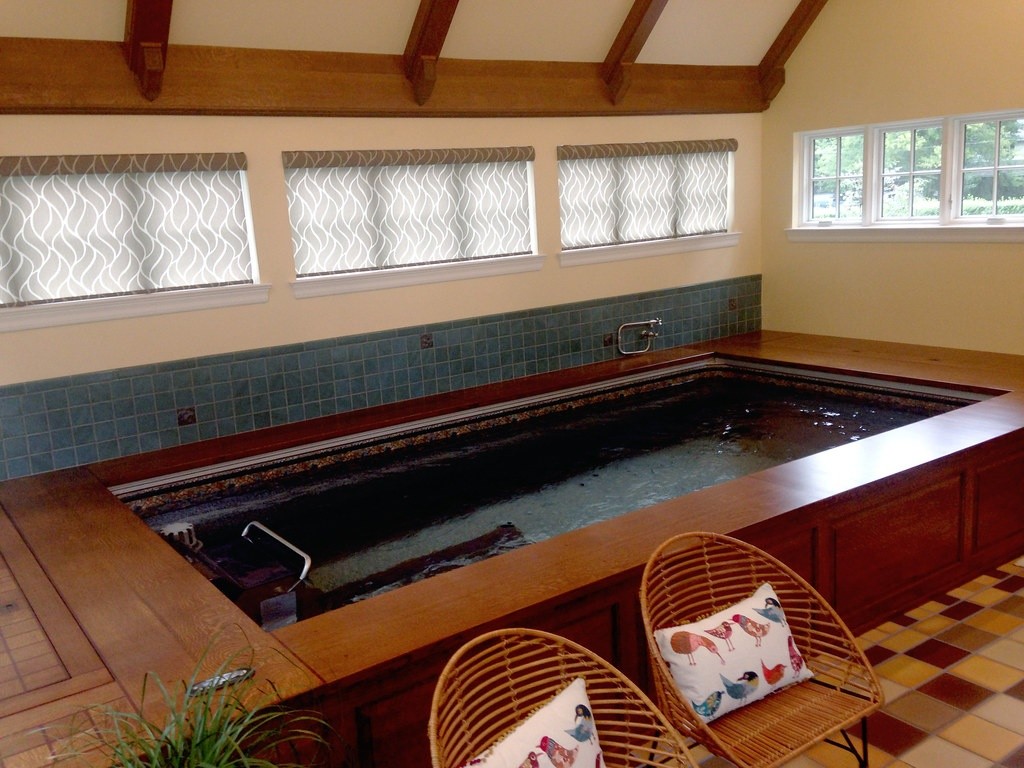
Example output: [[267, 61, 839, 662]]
[[426, 628, 701, 768], [638, 530, 886, 768]]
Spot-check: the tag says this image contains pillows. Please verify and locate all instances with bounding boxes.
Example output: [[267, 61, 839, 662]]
[[653, 580, 815, 732], [464, 674, 607, 768]]
